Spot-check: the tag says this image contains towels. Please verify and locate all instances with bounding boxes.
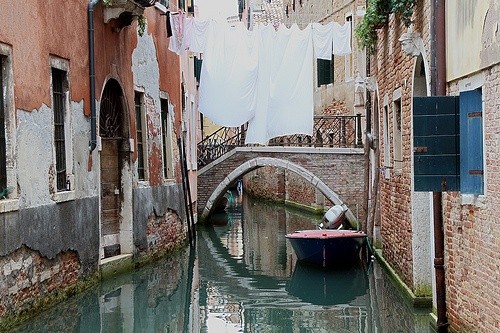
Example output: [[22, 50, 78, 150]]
[[333, 22, 352, 56], [312, 21, 333, 61], [164, 13, 206, 56], [196, 19, 259, 127], [244, 22, 314, 145]]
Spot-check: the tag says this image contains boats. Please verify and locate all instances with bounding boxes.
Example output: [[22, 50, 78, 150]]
[[285, 202, 369, 259]]
